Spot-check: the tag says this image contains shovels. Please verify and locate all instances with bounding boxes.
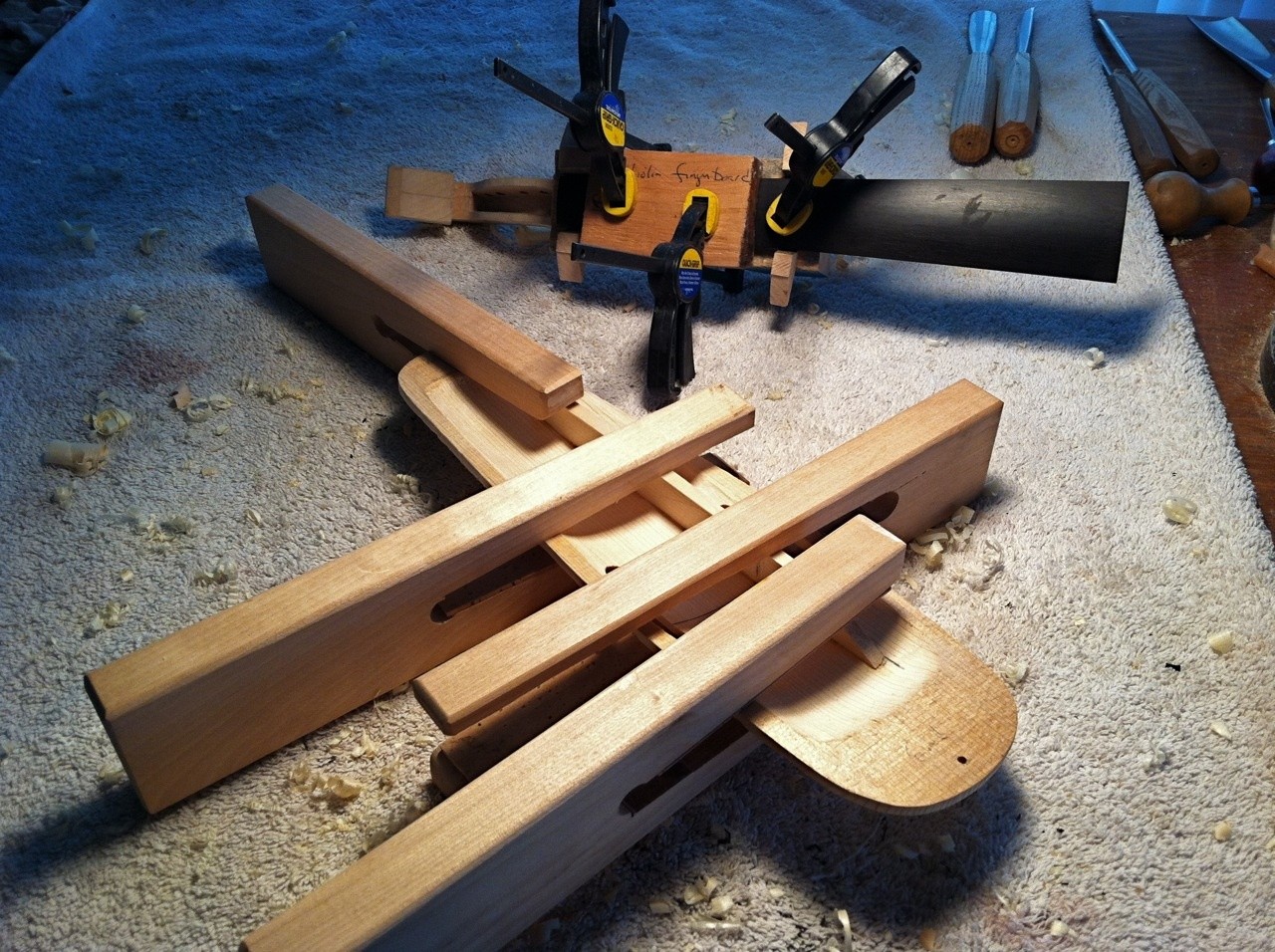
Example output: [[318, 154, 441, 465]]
[[1186, 15, 1275, 78]]
